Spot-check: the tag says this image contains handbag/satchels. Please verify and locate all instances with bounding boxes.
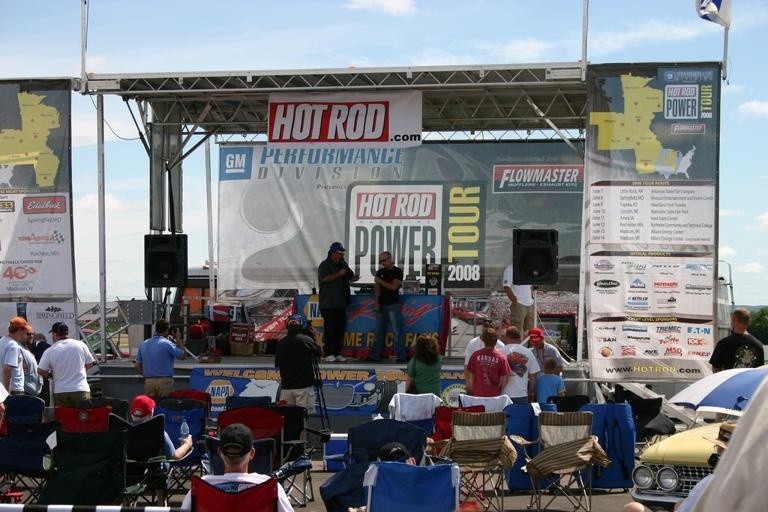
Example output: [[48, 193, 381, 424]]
[[406, 356, 418, 395]]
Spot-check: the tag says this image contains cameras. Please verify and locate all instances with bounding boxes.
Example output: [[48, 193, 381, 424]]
[[169, 325, 177, 335]]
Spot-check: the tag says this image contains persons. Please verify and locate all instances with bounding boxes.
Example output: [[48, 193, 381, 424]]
[[129, 394, 193, 460], [1, 316, 33, 397], [364, 251, 408, 364], [466, 327, 511, 398], [465, 320, 502, 368], [134, 319, 185, 400], [316, 241, 364, 365], [377, 441, 416, 466], [707, 307, 764, 373], [503, 264, 534, 343], [181, 422, 294, 512], [497, 326, 540, 406], [274, 320, 319, 418], [535, 359, 566, 405], [526, 328, 564, 376], [20, 326, 43, 397], [36, 322, 97, 407], [403, 332, 444, 400]]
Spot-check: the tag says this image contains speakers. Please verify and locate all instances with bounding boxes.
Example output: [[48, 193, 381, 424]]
[[143, 233, 188, 289], [511, 227, 558, 286]]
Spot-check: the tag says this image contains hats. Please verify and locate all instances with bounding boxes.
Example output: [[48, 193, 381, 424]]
[[330, 242, 346, 252], [220, 423, 253, 456], [528, 329, 544, 342], [379, 442, 411, 463], [131, 396, 156, 418], [49, 322, 68, 334], [9, 317, 32, 331], [286, 314, 305, 327]]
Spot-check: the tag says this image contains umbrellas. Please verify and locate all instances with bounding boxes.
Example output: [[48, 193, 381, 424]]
[[660, 366, 767, 418]]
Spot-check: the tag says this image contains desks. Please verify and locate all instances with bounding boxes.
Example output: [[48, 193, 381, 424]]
[[292, 294, 451, 362]]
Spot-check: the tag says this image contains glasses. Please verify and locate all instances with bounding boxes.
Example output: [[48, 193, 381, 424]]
[[27, 333, 33, 335], [380, 256, 391, 262]]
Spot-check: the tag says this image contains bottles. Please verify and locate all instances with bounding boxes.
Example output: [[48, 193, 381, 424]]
[[180, 419, 190, 440]]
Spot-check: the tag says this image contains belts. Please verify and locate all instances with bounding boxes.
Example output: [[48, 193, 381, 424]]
[[147, 376, 171, 379]]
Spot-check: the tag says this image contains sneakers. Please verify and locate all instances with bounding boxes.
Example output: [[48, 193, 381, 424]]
[[365, 357, 377, 363], [325, 355, 337, 363], [335, 356, 347, 362]]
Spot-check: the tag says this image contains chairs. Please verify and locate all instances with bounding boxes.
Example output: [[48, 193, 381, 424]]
[[524, 410, 611, 511], [215, 406, 285, 493], [579, 403, 636, 493], [190, 474, 283, 512], [344, 462, 462, 512], [499, 402, 563, 495], [547, 395, 590, 415], [433, 405, 486, 501], [255, 404, 315, 508], [224, 395, 273, 412], [118, 411, 193, 507], [387, 392, 443, 429], [168, 388, 213, 411], [159, 398, 210, 426], [205, 435, 277, 482], [456, 394, 514, 414], [1, 393, 56, 502], [618, 398, 665, 452], [54, 404, 115, 448], [346, 419, 434, 472], [441, 410, 518, 511]]
